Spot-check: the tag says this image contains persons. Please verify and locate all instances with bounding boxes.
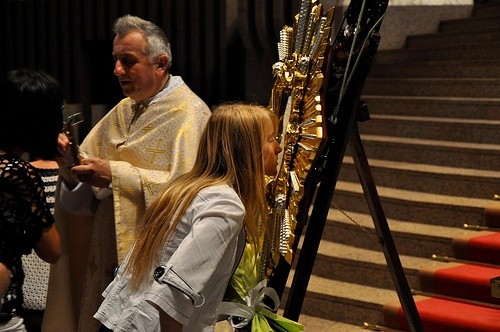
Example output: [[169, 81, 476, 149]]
[[41, 14, 212, 332], [0, 68, 65, 332], [93, 104, 282, 332]]
